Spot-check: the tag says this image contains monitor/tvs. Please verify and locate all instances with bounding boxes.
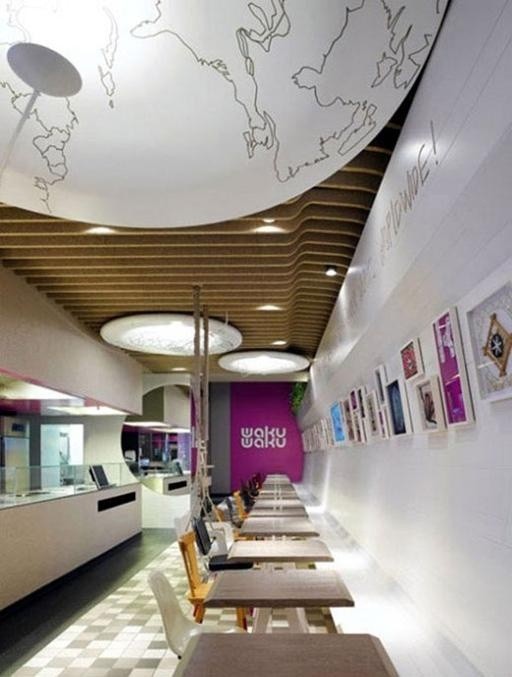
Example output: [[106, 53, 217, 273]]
[[89, 464, 109, 489]]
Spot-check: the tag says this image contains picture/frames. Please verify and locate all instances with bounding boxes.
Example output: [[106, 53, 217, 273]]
[[327, 271, 512, 447]]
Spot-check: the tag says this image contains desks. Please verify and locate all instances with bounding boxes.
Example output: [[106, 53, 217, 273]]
[[172, 473, 401, 677]]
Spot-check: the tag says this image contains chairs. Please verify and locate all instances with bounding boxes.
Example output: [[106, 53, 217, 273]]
[[147, 469, 264, 660]]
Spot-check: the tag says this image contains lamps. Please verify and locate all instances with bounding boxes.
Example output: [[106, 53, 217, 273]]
[[98, 310, 312, 378]]
